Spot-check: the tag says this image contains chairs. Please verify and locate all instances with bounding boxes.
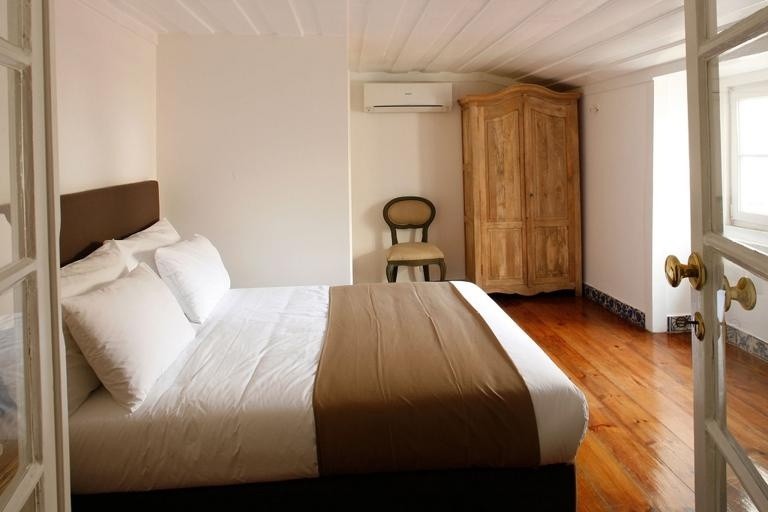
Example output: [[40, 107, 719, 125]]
[[383, 196, 447, 283]]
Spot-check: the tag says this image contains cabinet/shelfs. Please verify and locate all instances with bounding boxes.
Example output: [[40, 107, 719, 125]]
[[458, 85, 583, 298]]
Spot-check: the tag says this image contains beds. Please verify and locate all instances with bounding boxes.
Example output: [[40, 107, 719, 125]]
[[0, 181, 588, 512]]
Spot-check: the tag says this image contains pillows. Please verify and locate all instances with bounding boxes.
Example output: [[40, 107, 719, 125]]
[[104, 218, 182, 273], [61, 261, 198, 413], [0, 240, 131, 418], [154, 232, 231, 323]]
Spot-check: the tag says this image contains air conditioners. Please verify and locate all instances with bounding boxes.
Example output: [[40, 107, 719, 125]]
[[364, 82, 453, 113]]
